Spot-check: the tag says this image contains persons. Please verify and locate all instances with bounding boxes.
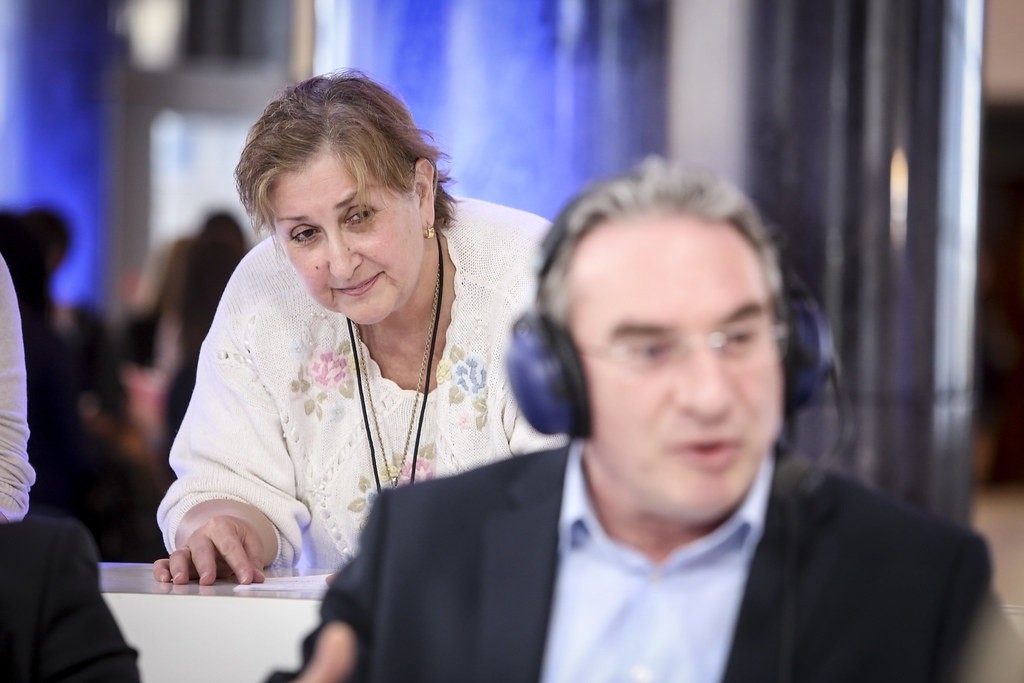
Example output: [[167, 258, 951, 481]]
[[155, 71, 578, 585], [0, 205, 255, 683], [265, 159, 1024, 683]]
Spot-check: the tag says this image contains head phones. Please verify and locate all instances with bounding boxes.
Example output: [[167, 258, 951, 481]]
[[506, 188, 832, 437]]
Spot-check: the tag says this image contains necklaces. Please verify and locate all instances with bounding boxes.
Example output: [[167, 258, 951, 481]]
[[348, 230, 443, 493]]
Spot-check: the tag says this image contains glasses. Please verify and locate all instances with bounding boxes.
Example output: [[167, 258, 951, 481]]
[[578, 314, 792, 397]]
